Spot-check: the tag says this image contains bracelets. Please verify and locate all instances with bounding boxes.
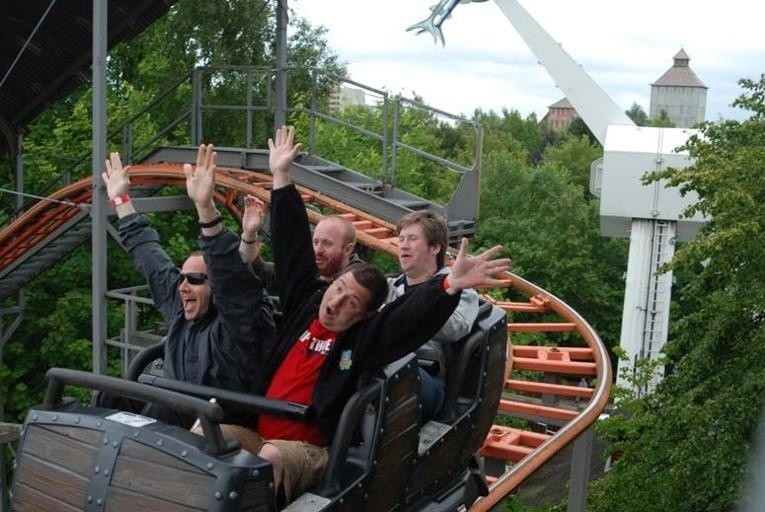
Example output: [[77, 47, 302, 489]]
[[240, 237, 256, 244], [108, 194, 129, 207], [196, 209, 224, 228]]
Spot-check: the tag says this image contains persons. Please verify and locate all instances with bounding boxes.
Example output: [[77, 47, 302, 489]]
[[236, 188, 368, 335], [365, 208, 482, 418], [189, 123, 511, 503], [100, 142, 275, 432]]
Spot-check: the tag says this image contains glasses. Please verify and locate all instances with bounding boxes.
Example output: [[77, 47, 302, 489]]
[[179, 273, 208, 285]]
